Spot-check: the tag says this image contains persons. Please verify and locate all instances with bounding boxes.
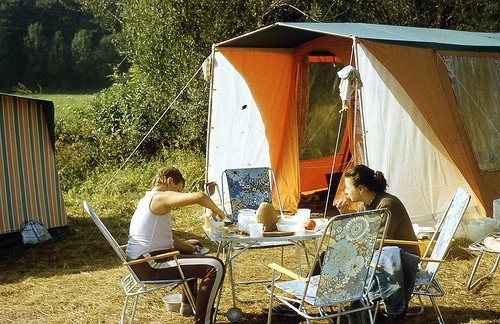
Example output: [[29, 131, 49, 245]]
[[126, 166, 226, 324], [344, 165, 421, 280]]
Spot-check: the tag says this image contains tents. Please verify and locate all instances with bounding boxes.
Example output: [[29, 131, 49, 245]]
[[203, 23, 500, 240], [0, 92, 67, 234]]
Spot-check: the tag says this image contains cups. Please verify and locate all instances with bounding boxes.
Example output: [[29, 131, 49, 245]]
[[297, 209, 310, 223], [250, 223, 266, 237], [211, 218, 224, 234]]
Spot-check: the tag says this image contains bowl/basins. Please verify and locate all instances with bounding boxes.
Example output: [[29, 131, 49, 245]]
[[162, 294, 182, 312], [276, 221, 299, 233]]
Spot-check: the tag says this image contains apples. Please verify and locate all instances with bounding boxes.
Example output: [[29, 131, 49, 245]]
[[304, 219, 316, 230]]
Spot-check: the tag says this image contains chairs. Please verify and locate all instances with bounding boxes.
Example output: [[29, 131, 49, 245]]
[[266, 207, 392, 324], [81, 199, 197, 324], [380, 186, 472, 324], [219, 166, 304, 318]]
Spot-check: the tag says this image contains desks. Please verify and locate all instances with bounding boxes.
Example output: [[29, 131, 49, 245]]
[[203, 218, 320, 324], [467, 232, 500, 290]]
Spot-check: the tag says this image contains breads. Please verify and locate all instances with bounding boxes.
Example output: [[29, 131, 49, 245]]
[[256, 202, 279, 232]]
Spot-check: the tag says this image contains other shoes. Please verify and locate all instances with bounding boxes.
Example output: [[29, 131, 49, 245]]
[[261, 305, 298, 317], [180, 305, 192, 316]]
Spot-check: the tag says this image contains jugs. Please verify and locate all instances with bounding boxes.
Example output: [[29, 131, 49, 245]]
[[238, 209, 258, 232]]
